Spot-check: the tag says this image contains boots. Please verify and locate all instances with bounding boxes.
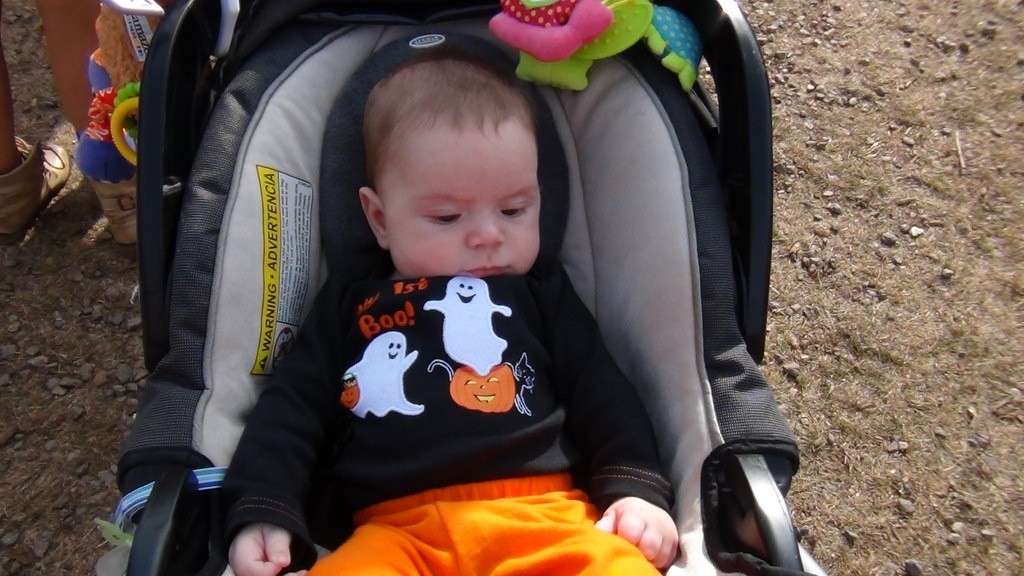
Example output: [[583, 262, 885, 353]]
[[0, 137, 71, 244]]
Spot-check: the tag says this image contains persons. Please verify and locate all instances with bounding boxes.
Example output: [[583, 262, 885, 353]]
[[225, 60, 677, 576], [0, 0, 139, 246]]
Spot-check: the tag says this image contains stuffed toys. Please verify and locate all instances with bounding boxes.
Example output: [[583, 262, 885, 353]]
[[490, 0, 702, 90], [75, 0, 164, 182]]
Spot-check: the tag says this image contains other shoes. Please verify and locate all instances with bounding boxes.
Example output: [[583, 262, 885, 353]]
[[89, 175, 137, 244]]
